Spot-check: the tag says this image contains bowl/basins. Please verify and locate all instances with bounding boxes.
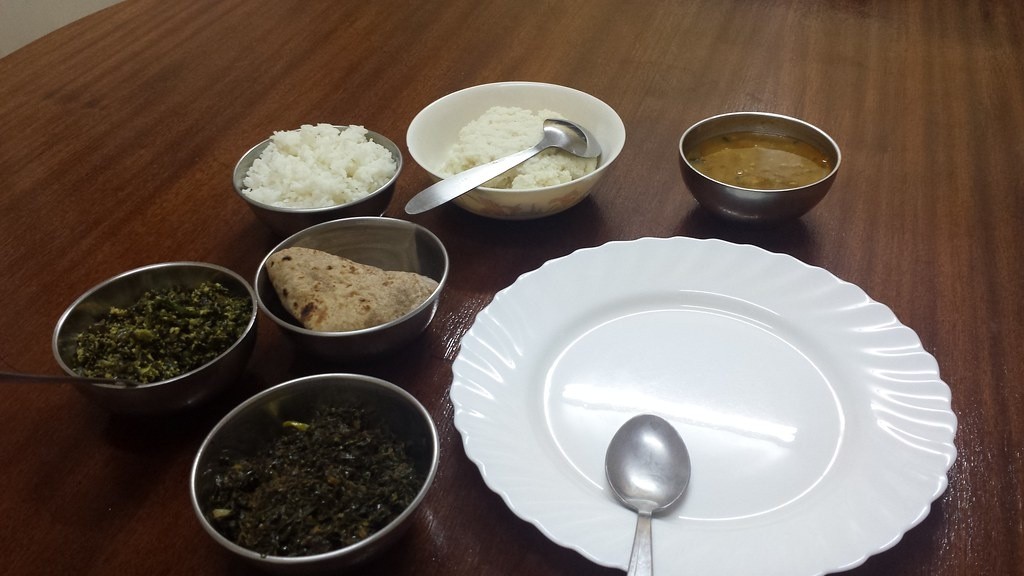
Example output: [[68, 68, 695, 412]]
[[680, 111, 842, 222], [233, 127, 403, 231], [255, 215, 450, 355], [52, 261, 255, 415], [406, 82, 626, 217], [189, 372, 439, 576]]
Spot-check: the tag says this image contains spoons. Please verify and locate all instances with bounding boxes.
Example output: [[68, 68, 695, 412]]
[[605, 414, 691, 576], [405, 118, 601, 216]]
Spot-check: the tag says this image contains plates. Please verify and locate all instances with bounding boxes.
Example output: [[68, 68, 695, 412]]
[[451, 237, 957, 576]]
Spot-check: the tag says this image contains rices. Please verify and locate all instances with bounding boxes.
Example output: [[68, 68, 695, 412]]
[[442, 107, 597, 189], [242, 124, 397, 209]]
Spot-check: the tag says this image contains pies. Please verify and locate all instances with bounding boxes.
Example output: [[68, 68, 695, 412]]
[[266, 246, 439, 331]]
